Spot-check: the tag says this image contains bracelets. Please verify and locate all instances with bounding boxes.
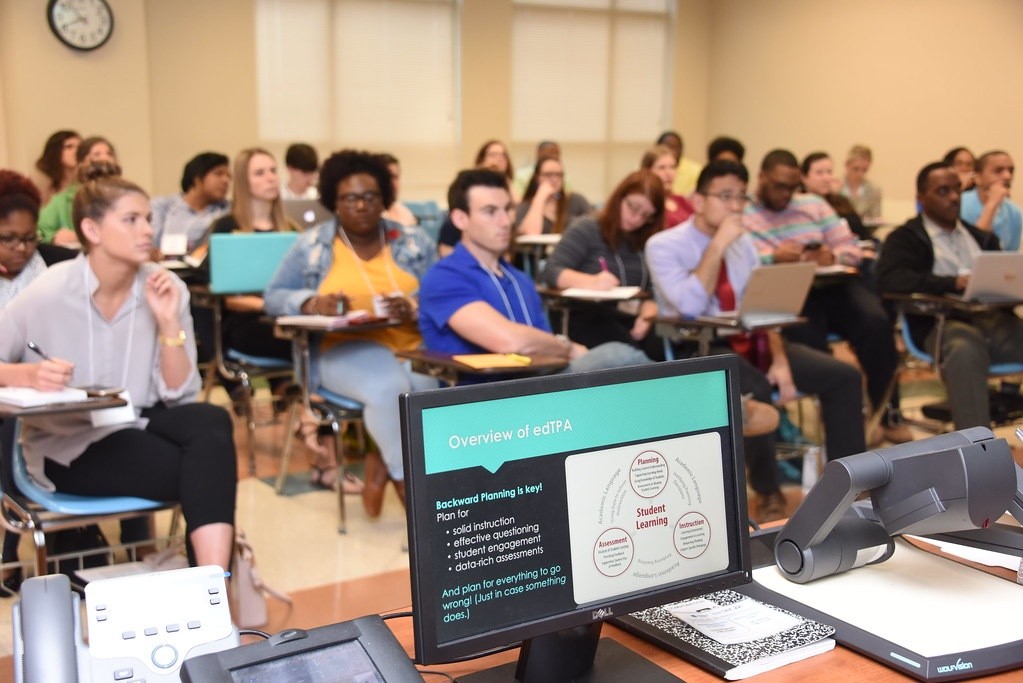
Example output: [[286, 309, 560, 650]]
[[159, 330, 186, 347]]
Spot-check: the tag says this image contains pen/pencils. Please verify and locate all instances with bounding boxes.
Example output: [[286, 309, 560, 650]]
[[599, 256, 608, 271], [28, 341, 51, 360], [0, 263, 8, 273], [336, 289, 344, 318]]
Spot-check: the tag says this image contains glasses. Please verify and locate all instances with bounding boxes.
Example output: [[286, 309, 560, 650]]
[[336, 192, 383, 209], [624, 195, 654, 223], [704, 191, 749, 203], [0, 228, 46, 250]]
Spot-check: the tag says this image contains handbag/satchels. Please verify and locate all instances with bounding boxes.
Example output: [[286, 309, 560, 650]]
[[151, 532, 269, 629]]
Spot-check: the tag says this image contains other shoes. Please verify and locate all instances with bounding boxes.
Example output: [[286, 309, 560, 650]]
[[741, 394, 780, 438], [757, 493, 789, 522], [887, 422, 912, 445]]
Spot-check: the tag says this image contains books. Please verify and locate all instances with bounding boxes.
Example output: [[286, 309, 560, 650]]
[[0, 385, 87, 408], [605, 589, 836, 680]]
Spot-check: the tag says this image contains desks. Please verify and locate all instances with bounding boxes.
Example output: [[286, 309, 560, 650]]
[[0, 446, 1023, 683]]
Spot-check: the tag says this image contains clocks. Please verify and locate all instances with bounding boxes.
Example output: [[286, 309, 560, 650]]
[[46, 0, 114, 50]]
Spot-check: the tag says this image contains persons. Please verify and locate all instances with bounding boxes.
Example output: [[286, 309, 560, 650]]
[[543, 172, 665, 363], [148, 152, 233, 261], [643, 160, 866, 524], [276, 144, 320, 201], [0, 159, 239, 569], [378, 153, 419, 226], [35, 130, 116, 245], [0, 169, 47, 309], [646, 131, 883, 233], [417, 169, 653, 374], [212, 147, 365, 495], [264, 149, 442, 505], [741, 148, 912, 444], [437, 140, 596, 256], [943, 149, 1023, 250], [876, 161, 1023, 429]]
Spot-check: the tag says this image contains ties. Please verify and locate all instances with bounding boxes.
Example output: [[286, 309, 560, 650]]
[[714, 257, 751, 357]]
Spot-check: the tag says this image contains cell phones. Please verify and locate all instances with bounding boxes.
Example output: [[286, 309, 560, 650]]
[[81, 385, 124, 395], [804, 241, 825, 252]]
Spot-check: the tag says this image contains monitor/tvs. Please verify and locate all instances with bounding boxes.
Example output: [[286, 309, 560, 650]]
[[399, 351, 752, 683]]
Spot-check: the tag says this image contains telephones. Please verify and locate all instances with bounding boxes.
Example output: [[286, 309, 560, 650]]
[[11, 564, 240, 683]]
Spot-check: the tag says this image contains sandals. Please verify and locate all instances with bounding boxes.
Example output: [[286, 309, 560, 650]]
[[310, 463, 363, 494], [293, 419, 327, 459]]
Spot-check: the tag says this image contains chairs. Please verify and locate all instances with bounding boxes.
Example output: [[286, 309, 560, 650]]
[[0, 204, 876, 602]]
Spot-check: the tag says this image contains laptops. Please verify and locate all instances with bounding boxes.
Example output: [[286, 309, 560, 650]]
[[207, 231, 302, 294], [943, 253, 1023, 302], [695, 260, 820, 325]]
